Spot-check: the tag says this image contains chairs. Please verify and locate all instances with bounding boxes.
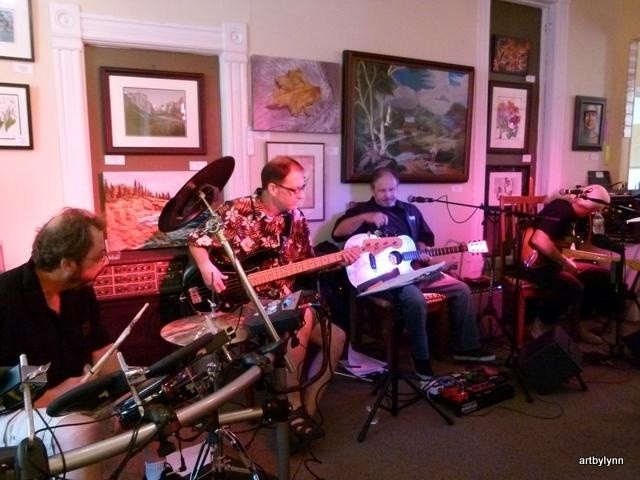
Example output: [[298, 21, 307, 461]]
[[350, 201, 450, 370], [497, 195, 552, 349]]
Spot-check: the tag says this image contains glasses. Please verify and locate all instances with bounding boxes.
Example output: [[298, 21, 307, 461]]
[[275, 182, 306, 195], [79, 252, 108, 265]]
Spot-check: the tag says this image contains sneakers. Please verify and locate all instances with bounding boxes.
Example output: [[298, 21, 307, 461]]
[[410, 355, 435, 381], [453, 345, 497, 363], [142, 466, 184, 480], [285, 404, 313, 436]]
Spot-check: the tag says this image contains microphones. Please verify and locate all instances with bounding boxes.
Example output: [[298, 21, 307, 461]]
[[407, 194, 434, 203], [558, 187, 591, 195]]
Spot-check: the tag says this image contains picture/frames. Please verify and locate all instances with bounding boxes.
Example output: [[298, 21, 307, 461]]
[[100, 66, 207, 155], [491, 34, 531, 76], [572, 95, 607, 151], [0, 0, 35, 62], [0, 83, 34, 150], [485, 165, 530, 215], [265, 141, 326, 223], [488, 80, 533, 155], [340, 49, 475, 184]]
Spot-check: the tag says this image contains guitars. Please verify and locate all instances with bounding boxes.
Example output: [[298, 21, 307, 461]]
[[344, 231, 490, 295], [178, 230, 403, 317], [519, 225, 640, 276]]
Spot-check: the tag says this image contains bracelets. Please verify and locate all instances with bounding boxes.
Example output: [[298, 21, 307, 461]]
[[560, 258, 568, 267]]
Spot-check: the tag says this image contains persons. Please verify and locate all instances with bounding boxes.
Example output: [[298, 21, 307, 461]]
[[186, 154, 346, 443], [517, 183, 623, 345], [331, 169, 495, 380], [580, 104, 599, 144], [0, 207, 142, 480]]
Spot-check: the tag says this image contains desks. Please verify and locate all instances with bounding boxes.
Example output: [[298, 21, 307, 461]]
[[609, 193, 640, 242]]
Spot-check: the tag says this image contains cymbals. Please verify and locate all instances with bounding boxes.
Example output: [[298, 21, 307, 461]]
[[160, 311, 258, 349], [158, 155, 236, 233]]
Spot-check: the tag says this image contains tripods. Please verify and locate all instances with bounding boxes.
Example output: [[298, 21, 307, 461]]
[[452, 216, 522, 358], [582, 226, 640, 369], [480, 218, 540, 403], [354, 264, 455, 444]]
